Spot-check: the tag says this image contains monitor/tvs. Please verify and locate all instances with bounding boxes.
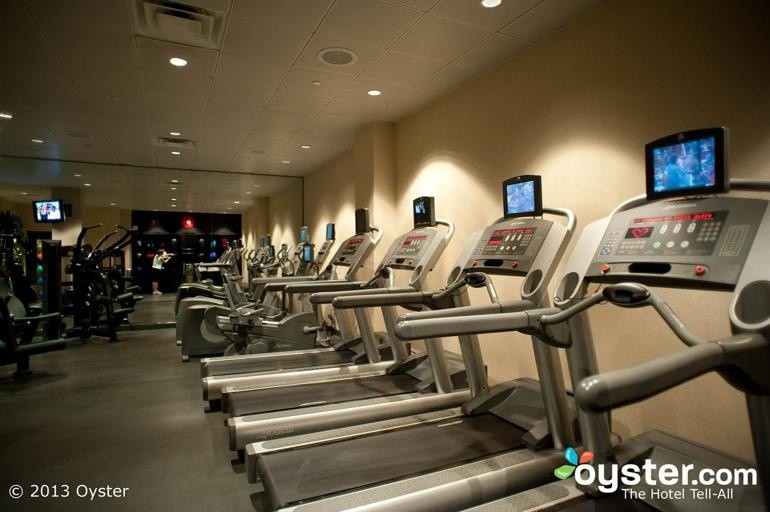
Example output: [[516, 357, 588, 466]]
[[32, 199, 65, 223], [355, 208, 369, 233], [503, 173, 543, 219], [258, 222, 336, 263], [643, 127, 729, 200], [413, 195, 435, 226]]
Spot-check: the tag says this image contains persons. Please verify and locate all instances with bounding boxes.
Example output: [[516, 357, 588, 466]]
[[418, 201, 423, 213], [509, 183, 533, 212], [415, 202, 419, 213], [49, 206, 60, 218], [662, 153, 693, 190], [151, 248, 177, 295], [39, 204, 48, 220]]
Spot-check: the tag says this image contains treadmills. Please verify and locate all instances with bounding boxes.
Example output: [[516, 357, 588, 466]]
[[203, 180, 768, 512]]
